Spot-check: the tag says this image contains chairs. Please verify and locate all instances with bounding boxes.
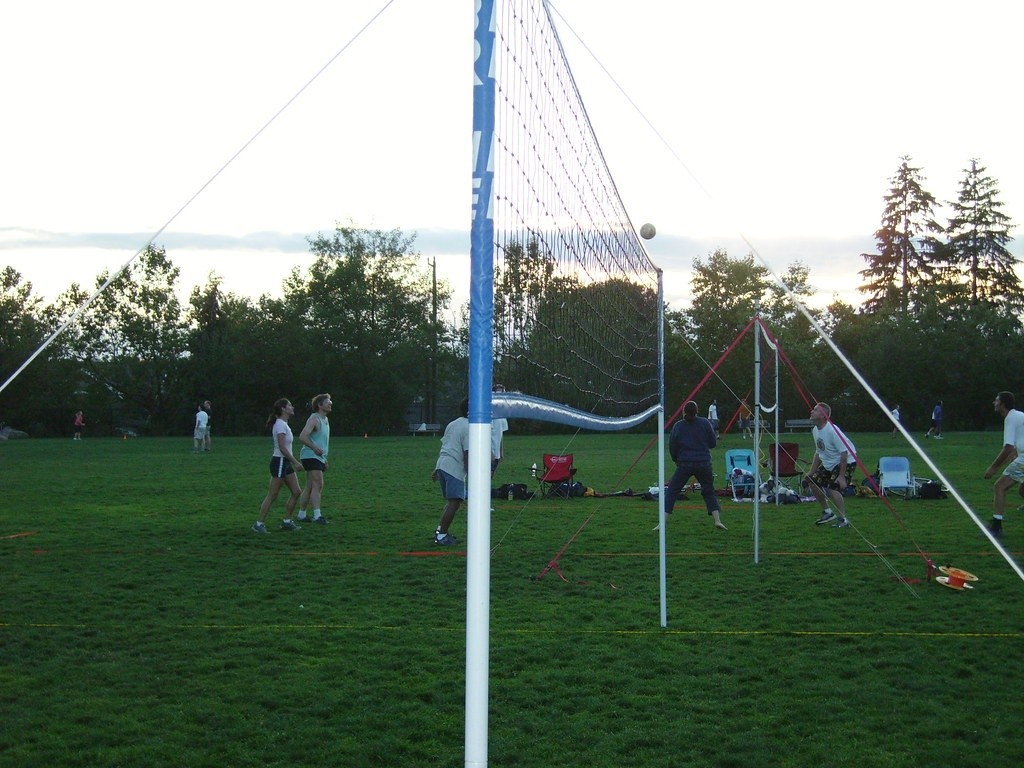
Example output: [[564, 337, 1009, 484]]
[[877, 456, 914, 498], [763, 443, 812, 494], [725, 448, 762, 496], [529, 453, 577, 499]]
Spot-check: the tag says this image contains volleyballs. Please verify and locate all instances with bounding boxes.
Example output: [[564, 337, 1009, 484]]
[[640, 222, 656, 239]]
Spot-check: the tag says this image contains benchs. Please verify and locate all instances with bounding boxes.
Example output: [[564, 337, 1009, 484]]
[[738, 418, 770, 432], [785, 418, 816, 432], [408, 423, 440, 437]]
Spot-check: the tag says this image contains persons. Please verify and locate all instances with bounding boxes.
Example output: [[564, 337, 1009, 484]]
[[740, 398, 753, 439], [251, 399, 304, 533], [803, 402, 856, 527], [74, 411, 85, 440], [708, 400, 720, 440], [891, 405, 902, 438], [491, 384, 508, 515], [193, 400, 213, 453], [295, 393, 333, 524], [652, 401, 728, 530], [431, 398, 469, 546], [926, 400, 945, 439], [983, 392, 1024, 539]]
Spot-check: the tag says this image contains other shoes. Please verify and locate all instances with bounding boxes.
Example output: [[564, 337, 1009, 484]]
[[314, 517, 327, 524], [986, 518, 1003, 537], [296, 517, 312, 522], [934, 435, 943, 439], [925, 434, 927, 439], [435, 530, 458, 545]]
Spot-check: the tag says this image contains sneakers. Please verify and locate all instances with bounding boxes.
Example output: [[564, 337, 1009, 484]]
[[280, 521, 298, 531], [831, 517, 847, 527], [816, 511, 836, 524], [251, 522, 271, 533]]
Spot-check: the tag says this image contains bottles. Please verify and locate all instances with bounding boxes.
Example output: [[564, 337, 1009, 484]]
[[531, 462, 537, 477], [508, 489, 514, 500]]
